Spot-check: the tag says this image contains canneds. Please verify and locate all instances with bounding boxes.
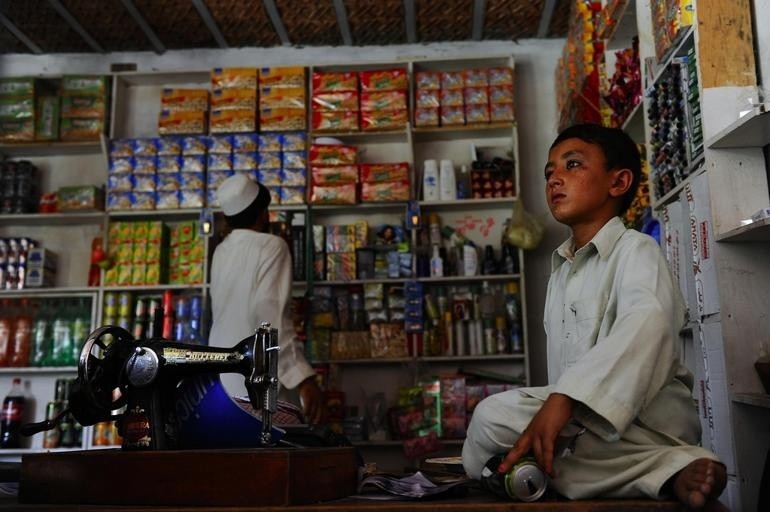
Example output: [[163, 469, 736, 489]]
[[100, 291, 201, 359], [481, 450, 548, 502], [88, 238, 103, 287], [43, 377, 83, 447], [92, 421, 123, 445]]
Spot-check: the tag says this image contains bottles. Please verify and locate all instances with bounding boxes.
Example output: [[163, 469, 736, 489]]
[[501, 242, 517, 274], [1, 296, 91, 369], [427, 213, 441, 249], [481, 244, 498, 275], [0, 377, 26, 451], [86, 221, 104, 287], [438, 159, 457, 201], [423, 158, 440, 203], [429, 244, 464, 278], [440, 225, 473, 249], [421, 280, 524, 356]]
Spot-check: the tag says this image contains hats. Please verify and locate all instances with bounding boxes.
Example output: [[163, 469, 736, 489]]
[[216, 172, 260, 217]]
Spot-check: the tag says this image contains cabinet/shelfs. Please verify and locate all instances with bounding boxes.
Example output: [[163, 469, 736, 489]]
[[2, 55, 531, 456], [601, 0, 768, 509]]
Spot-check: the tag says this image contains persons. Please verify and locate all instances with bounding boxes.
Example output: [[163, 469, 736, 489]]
[[462, 124, 727, 512], [207, 172, 326, 425]]
